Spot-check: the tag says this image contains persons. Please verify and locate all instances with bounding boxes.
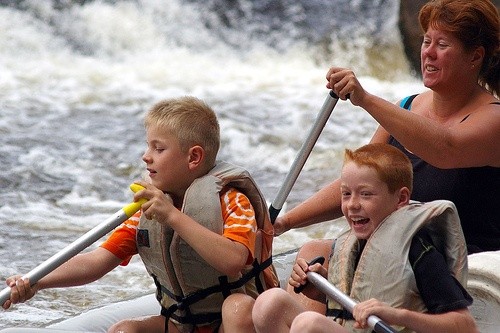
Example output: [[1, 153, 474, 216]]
[[251, 141, 480, 333], [271, 0, 500, 301], [1, 97, 280, 333]]
